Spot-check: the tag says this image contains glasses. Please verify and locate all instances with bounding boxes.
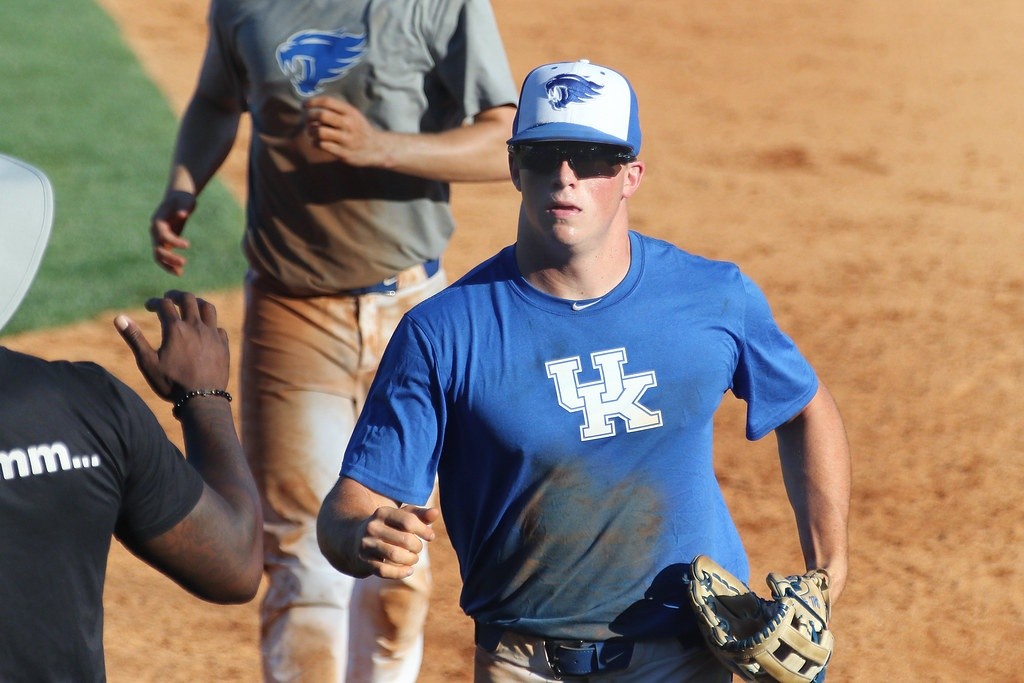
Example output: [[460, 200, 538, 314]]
[[508, 139, 637, 179]]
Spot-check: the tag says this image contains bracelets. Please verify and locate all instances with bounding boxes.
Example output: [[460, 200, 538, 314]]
[[171, 389, 232, 421]]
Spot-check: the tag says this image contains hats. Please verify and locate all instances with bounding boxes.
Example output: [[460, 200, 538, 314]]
[[507, 59, 641, 157], [0, 153, 53, 329]]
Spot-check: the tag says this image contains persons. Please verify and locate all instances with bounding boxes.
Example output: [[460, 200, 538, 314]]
[[149, 1, 521, 683], [0, 151, 265, 683], [316, 57, 852, 683]]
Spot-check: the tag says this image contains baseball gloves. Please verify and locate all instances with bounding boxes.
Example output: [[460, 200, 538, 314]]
[[682, 551, 836, 683]]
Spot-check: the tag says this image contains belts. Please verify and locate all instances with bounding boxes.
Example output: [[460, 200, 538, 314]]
[[247, 258, 439, 297], [475, 620, 702, 677]]
